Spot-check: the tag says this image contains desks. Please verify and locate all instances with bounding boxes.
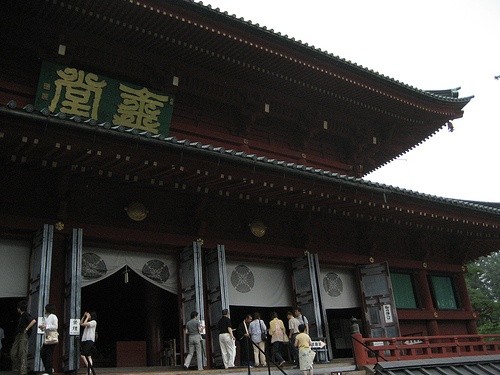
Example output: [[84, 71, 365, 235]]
[[114, 340, 147, 366]]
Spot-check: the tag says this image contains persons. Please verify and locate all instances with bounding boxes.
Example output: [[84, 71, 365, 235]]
[[287, 312, 300, 369], [294, 307, 309, 334], [184, 311, 203, 371], [249, 313, 267, 368], [39, 303, 58, 375], [269, 311, 286, 367], [295, 324, 314, 375], [80, 312, 97, 373], [237, 315, 254, 365], [218, 309, 236, 369], [10, 302, 37, 375]]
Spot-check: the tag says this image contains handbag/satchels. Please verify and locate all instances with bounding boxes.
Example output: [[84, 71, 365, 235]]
[[261, 334, 268, 341], [44, 328, 59, 344]]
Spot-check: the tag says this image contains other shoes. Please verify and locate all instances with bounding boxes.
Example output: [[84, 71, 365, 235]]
[[229, 366, 237, 369], [279, 361, 286, 368], [42, 373, 53, 375], [262, 364, 267, 367], [255, 365, 259, 367], [184, 365, 188, 370], [293, 365, 300, 368]]
